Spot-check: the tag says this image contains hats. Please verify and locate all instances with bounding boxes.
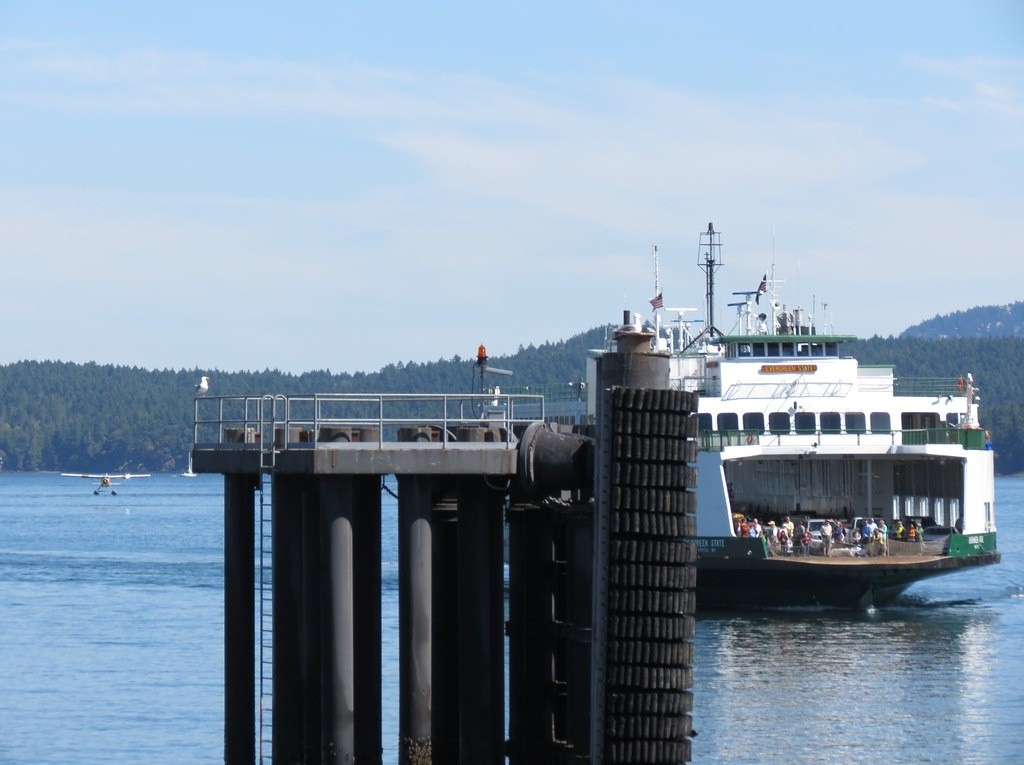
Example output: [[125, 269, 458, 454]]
[[768, 521, 775, 524], [826, 519, 830, 522]]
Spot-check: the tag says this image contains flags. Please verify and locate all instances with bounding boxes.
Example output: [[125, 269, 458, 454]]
[[755, 274, 768, 305], [649, 292, 664, 312]]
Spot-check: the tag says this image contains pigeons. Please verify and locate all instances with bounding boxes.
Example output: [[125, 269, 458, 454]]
[[193, 376, 209, 397]]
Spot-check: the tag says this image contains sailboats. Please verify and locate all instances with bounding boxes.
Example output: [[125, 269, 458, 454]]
[[180, 451, 197, 477]]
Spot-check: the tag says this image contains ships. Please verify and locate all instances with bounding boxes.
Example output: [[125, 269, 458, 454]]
[[471, 221, 1001, 609]]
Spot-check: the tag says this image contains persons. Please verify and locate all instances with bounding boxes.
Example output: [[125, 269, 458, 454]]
[[738, 517, 925, 556]]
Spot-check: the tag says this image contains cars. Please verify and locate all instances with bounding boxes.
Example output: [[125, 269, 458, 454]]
[[924, 526, 962, 554], [790, 516, 849, 556], [852, 516, 886, 532], [902, 516, 937, 542]]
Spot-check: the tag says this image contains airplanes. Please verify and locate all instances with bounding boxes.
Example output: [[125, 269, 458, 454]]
[[59, 470, 151, 497]]
